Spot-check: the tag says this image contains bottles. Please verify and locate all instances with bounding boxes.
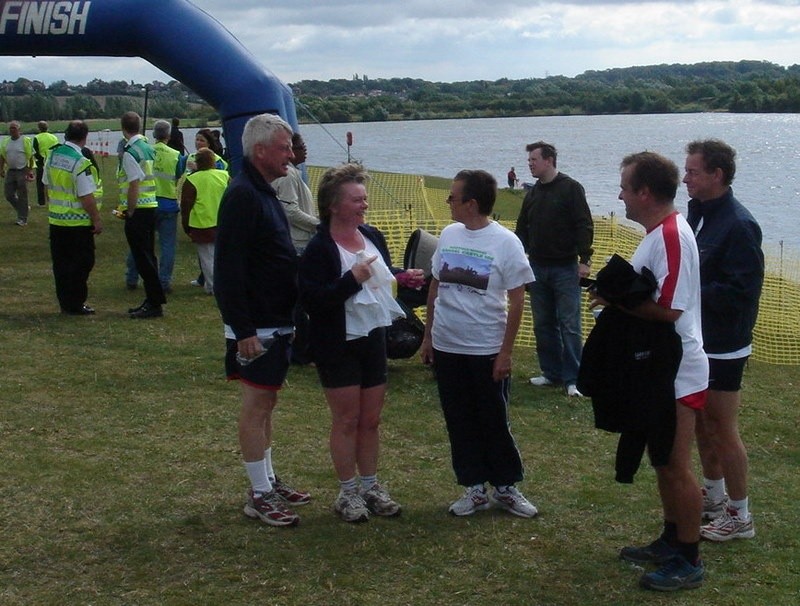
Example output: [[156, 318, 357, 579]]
[[357, 249, 386, 290], [236, 330, 280, 366]]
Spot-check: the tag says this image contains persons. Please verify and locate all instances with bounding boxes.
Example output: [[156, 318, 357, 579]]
[[683, 140, 767, 543], [586, 152, 710, 591], [508, 167, 518, 191], [514, 140, 595, 398], [33, 111, 232, 319], [270, 133, 322, 256], [295, 159, 428, 524], [420, 170, 541, 520], [213, 111, 312, 527], [0, 119, 33, 228]]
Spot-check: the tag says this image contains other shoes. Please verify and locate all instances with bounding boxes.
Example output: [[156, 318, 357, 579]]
[[128, 299, 149, 313], [14, 217, 27, 226], [66, 304, 96, 315], [190, 279, 201, 285], [130, 302, 163, 318], [164, 287, 172, 292]]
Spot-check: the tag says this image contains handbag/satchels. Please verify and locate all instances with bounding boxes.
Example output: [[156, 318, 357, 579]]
[[384, 296, 423, 359]]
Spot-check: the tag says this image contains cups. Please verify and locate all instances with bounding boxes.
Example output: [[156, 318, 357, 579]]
[[589, 301, 605, 319], [186, 160, 193, 174]]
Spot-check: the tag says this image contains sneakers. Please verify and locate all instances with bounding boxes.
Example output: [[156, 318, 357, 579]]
[[244, 487, 299, 527], [567, 385, 583, 397], [334, 486, 370, 521], [447, 487, 490, 516], [529, 376, 553, 386], [272, 474, 311, 505], [699, 486, 729, 519], [642, 552, 704, 591], [620, 524, 679, 563], [492, 487, 538, 519], [700, 501, 756, 541], [359, 483, 401, 517]]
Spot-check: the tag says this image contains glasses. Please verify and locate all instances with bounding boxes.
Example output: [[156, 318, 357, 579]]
[[448, 195, 474, 202], [292, 143, 305, 150]]
